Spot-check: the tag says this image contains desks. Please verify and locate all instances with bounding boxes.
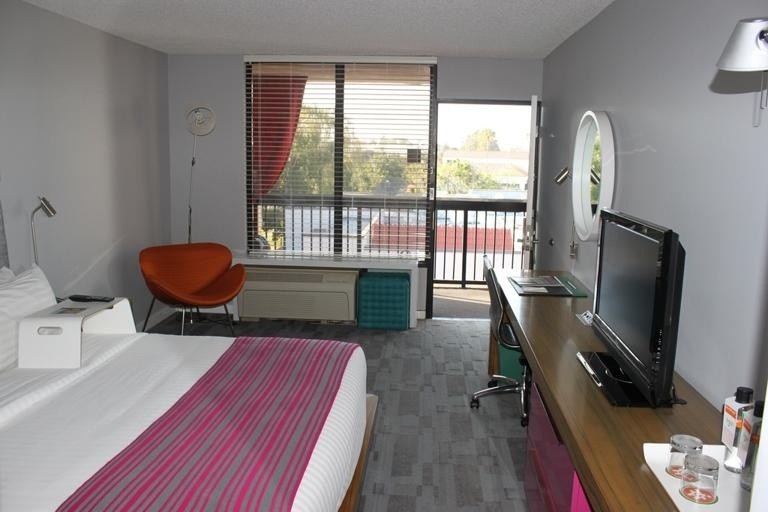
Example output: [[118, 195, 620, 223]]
[[232, 252, 419, 329], [481, 265, 767, 512], [17, 294, 137, 370]]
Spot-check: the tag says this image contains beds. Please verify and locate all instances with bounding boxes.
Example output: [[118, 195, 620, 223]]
[[0, 214, 379, 512]]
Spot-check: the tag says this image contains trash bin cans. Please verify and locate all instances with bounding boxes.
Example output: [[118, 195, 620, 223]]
[[496, 339, 524, 388]]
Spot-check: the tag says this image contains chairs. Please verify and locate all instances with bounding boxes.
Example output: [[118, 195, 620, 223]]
[[470, 253, 533, 425], [137, 241, 246, 338]]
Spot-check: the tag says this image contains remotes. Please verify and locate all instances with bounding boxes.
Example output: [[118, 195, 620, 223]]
[[69, 295, 114, 301]]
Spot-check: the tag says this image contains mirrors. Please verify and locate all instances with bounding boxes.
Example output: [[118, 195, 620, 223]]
[[572, 111, 619, 243]]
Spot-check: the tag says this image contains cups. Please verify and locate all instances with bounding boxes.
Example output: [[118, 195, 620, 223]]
[[666, 434, 704, 482], [680, 453, 720, 503]]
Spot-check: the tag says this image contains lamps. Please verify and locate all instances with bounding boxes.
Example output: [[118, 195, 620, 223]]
[[554, 167, 572, 186], [178, 105, 218, 245], [24, 196, 58, 269], [715, 17, 768, 112]]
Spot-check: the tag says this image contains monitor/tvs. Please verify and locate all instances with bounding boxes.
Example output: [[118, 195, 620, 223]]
[[576, 208, 684, 406]]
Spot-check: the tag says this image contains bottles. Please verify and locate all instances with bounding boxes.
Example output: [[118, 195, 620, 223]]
[[725, 386, 755, 474], [739, 400, 765, 492]]
